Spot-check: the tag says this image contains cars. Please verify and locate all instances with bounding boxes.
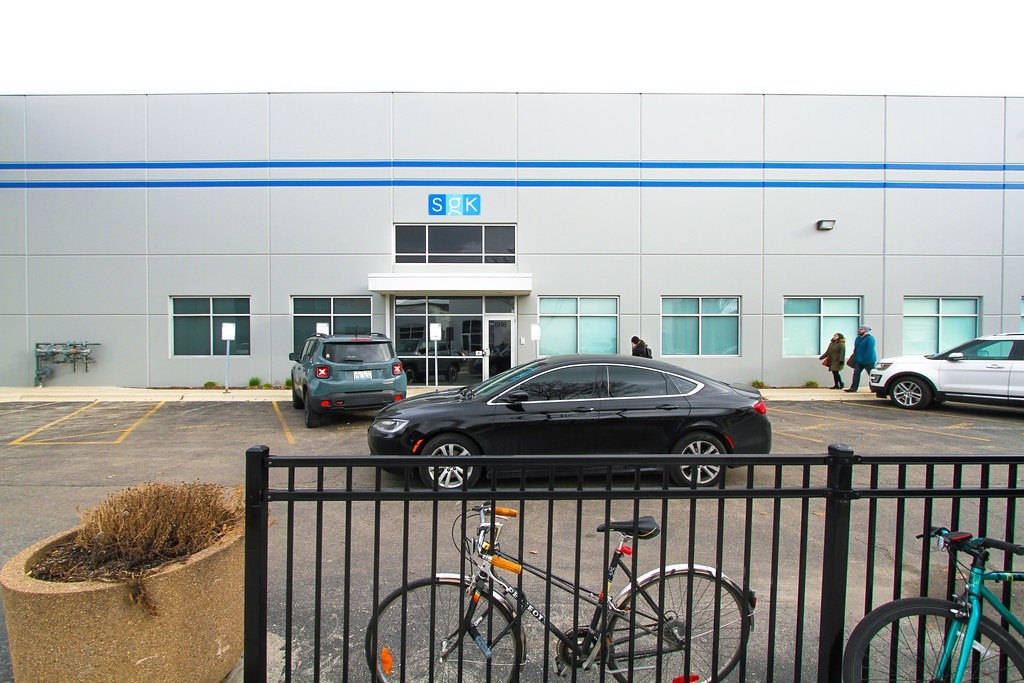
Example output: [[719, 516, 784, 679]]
[[367, 354, 773, 492], [467, 343, 511, 378]]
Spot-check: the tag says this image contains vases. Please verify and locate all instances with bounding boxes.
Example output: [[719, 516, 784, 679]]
[[0, 513, 245, 683]]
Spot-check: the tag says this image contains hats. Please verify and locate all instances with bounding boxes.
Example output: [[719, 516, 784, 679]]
[[859, 325, 871, 332], [838, 333, 844, 339]]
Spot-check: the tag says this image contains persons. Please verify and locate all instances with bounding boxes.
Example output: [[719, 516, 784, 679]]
[[631, 336, 647, 358], [844, 325, 877, 393], [819, 333, 845, 389]]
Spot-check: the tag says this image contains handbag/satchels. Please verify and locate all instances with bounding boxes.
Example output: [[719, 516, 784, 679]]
[[822, 356, 830, 366], [847, 354, 855, 368], [645, 345, 652, 359]]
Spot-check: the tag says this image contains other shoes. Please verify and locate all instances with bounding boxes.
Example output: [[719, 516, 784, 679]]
[[844, 388, 856, 392], [840, 383, 844, 389], [830, 385, 840, 389]]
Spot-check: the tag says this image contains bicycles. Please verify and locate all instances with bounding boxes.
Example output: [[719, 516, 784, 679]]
[[364, 500, 758, 683], [841, 525, 1023, 683]]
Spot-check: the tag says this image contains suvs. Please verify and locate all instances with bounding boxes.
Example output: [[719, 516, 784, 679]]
[[396, 340, 464, 384], [868, 332, 1024, 410], [288, 332, 408, 429]]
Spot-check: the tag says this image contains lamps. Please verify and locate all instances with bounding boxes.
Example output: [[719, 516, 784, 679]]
[[817, 220, 835, 230]]
